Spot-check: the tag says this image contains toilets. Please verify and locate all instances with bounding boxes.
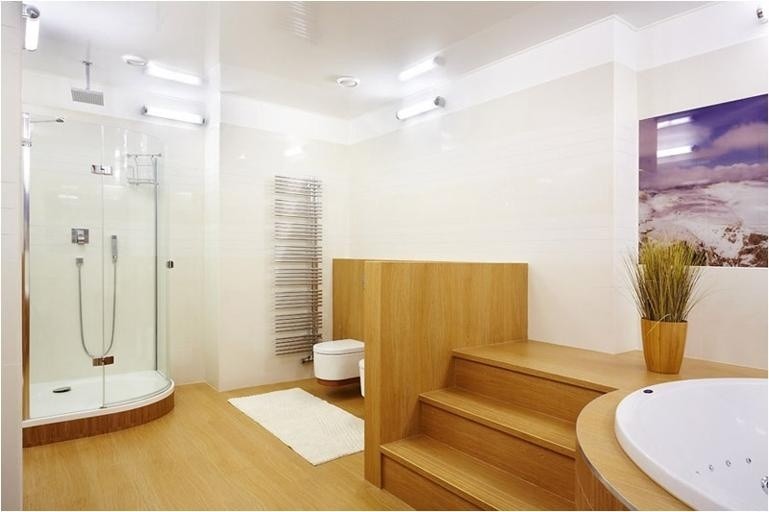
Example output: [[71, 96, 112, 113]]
[[358, 359, 365, 398], [312, 338, 365, 382]]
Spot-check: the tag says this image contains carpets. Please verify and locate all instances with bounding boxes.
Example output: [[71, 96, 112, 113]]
[[227, 386, 365, 466]]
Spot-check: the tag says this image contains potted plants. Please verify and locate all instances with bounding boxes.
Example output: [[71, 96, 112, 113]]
[[626, 235, 708, 377]]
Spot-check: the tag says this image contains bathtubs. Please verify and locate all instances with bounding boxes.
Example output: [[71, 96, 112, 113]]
[[614, 376, 768, 510]]
